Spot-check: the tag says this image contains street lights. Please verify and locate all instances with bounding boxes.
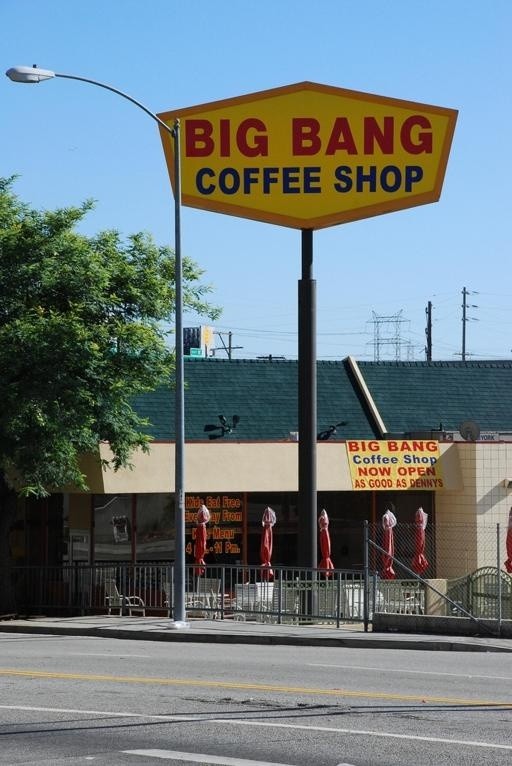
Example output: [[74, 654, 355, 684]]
[[4, 63, 192, 631]]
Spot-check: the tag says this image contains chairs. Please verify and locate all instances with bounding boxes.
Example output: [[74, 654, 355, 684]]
[[103, 578, 145, 617], [163, 578, 424, 625]]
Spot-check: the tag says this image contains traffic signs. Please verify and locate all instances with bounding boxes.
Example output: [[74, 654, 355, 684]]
[[190, 348, 203, 357]]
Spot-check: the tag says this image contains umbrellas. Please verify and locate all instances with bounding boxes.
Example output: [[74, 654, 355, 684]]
[[318, 507, 335, 587], [380, 508, 395, 580], [410, 505, 428, 590], [504, 505, 512, 575], [193, 504, 212, 595], [258, 504, 279, 602]]
